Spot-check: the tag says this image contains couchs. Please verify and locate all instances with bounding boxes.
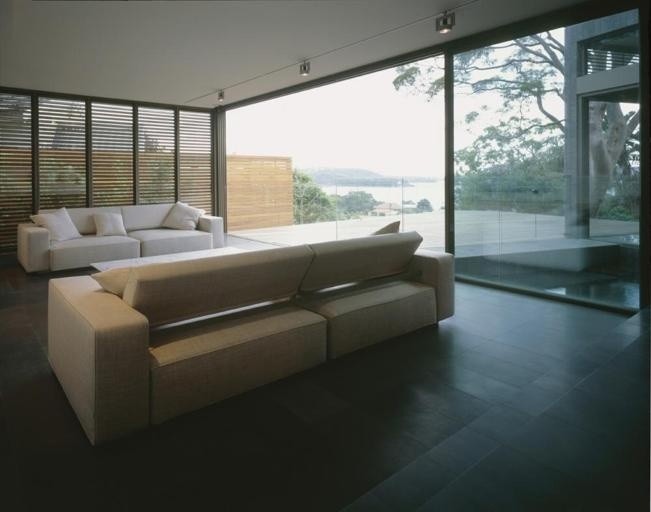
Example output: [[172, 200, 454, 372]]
[[49, 230, 455, 449], [17, 203, 224, 275]]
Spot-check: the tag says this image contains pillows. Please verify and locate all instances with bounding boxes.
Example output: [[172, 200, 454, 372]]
[[371, 220, 401, 235], [161, 202, 205, 231], [92, 212, 128, 238], [92, 266, 136, 298], [28, 207, 82, 243]]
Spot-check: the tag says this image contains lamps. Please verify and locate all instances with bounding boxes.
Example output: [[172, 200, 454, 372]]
[[435, 9, 456, 33], [299, 60, 311, 76]]
